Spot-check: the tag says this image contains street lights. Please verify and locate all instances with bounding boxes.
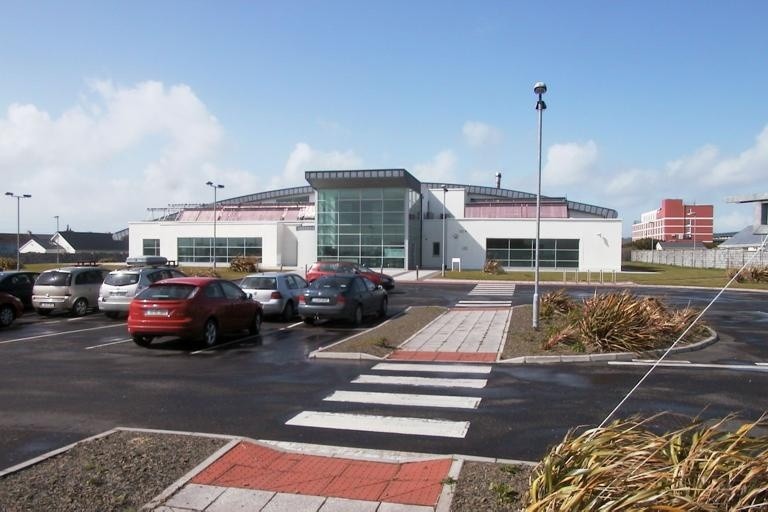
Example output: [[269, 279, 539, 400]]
[[687, 210, 696, 269], [205, 181, 224, 273], [530, 83, 548, 329], [440, 184, 448, 275], [649, 221, 654, 263], [5, 192, 33, 270], [54, 215, 61, 264]]
